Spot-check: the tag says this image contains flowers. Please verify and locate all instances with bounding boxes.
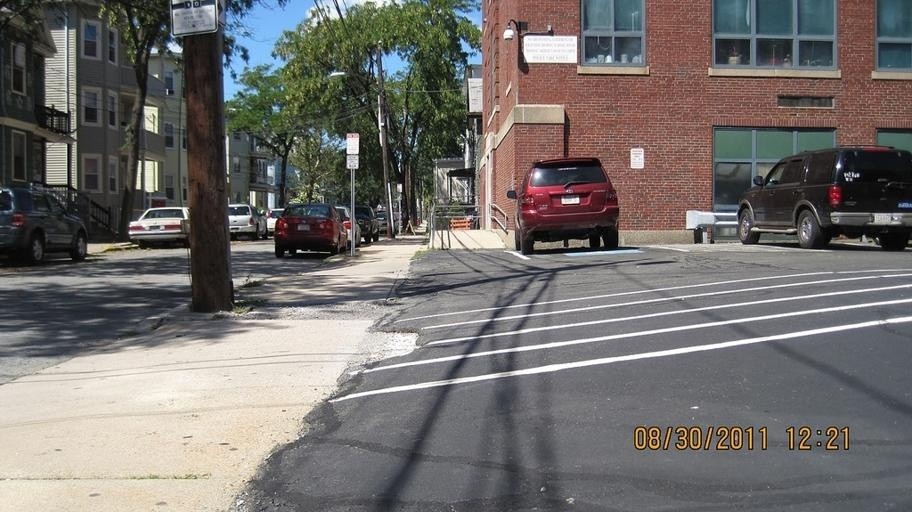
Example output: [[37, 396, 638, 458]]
[[727, 46, 742, 57]]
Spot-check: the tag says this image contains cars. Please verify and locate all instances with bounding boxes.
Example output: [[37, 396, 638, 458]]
[[228, 202, 421, 258], [128, 205, 191, 249]]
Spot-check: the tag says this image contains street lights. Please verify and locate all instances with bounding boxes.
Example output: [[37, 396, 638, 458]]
[[330, 70, 396, 240]]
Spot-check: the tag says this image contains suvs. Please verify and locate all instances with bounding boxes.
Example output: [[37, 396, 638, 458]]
[[0, 185, 91, 265], [505, 156, 621, 256], [733, 143, 912, 250]]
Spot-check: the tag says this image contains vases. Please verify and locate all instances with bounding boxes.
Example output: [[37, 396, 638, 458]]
[[727, 56, 741, 65]]
